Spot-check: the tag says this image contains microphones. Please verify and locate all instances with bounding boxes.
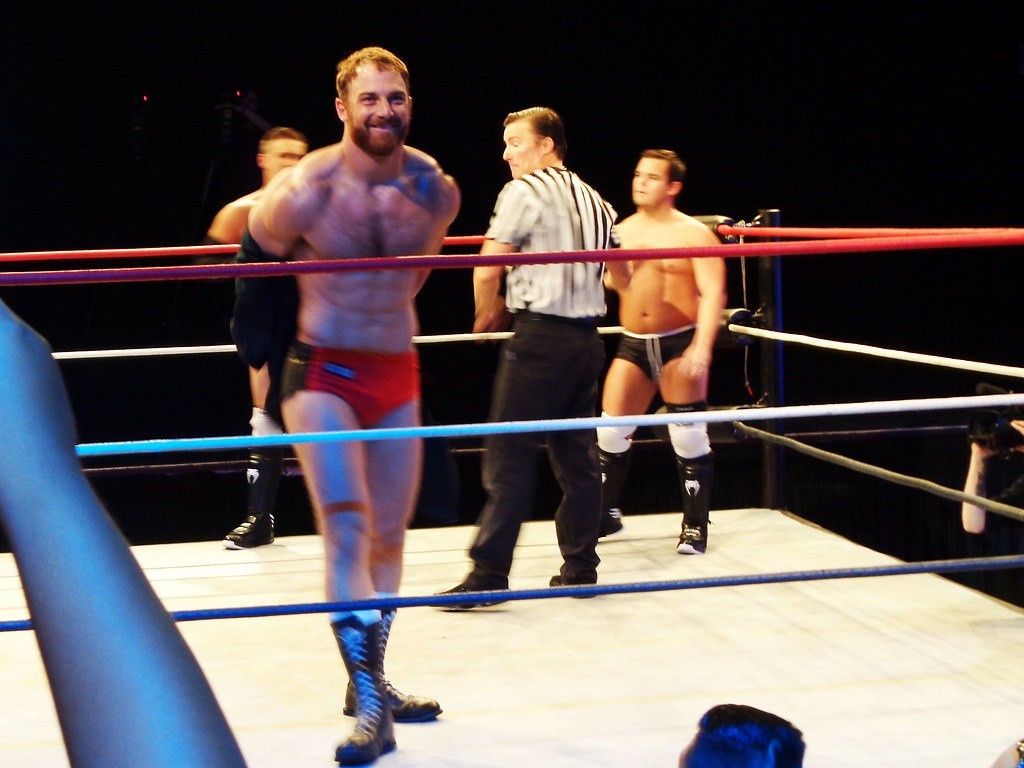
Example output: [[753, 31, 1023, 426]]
[[975, 383, 1013, 396]]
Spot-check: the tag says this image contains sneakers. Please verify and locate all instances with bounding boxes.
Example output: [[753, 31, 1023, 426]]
[[223, 513, 274, 550], [677, 512, 712, 554], [597, 508, 623, 536]]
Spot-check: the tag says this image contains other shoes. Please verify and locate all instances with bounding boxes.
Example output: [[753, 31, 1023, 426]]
[[433, 573, 508, 610], [550, 577, 595, 598]]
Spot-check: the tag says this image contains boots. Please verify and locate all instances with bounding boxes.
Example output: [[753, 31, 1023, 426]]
[[344, 607, 443, 723], [329, 613, 395, 764]]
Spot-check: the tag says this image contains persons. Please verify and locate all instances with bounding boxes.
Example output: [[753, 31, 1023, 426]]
[[679, 704, 805, 768], [429, 107, 617, 613], [189, 125, 309, 550], [595, 149, 725, 556], [232, 46, 460, 766]]
